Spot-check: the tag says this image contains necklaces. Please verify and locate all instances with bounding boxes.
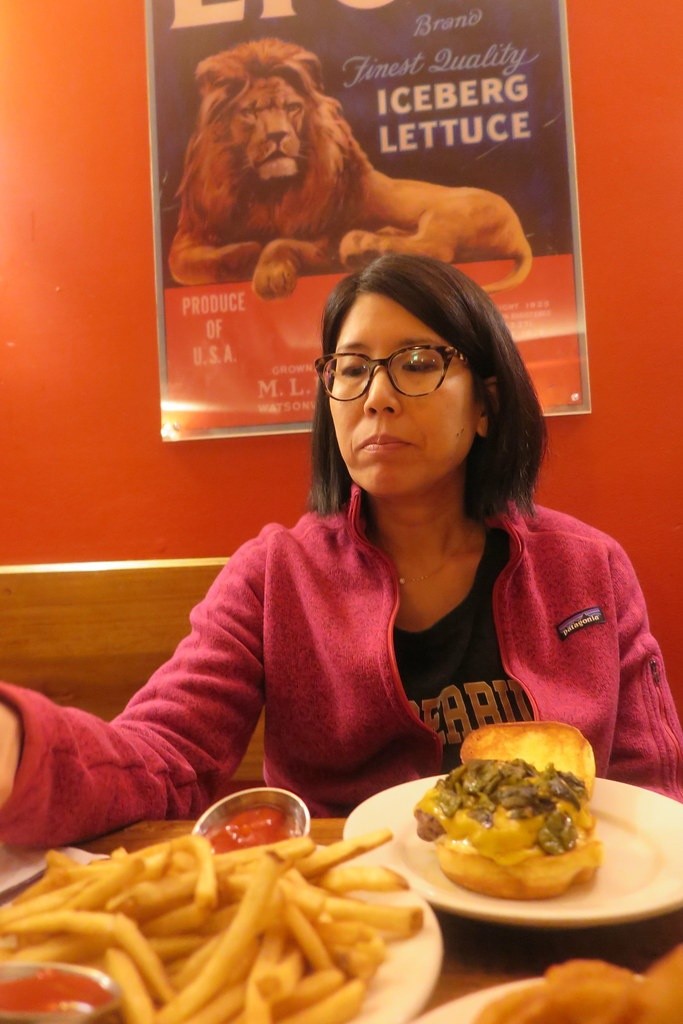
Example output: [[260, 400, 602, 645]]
[[399, 525, 475, 584]]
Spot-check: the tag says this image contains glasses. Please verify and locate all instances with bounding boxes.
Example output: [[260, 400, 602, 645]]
[[315, 344, 469, 401]]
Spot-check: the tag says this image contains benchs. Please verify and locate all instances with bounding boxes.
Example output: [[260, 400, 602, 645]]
[[2, 557, 266, 813]]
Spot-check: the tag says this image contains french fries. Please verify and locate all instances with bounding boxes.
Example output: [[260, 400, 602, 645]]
[[0, 825, 422, 1024]]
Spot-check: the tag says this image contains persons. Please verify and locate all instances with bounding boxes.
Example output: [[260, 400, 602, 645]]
[[0, 252, 683, 850]]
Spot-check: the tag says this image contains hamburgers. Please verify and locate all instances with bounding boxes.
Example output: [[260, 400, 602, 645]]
[[413, 721, 603, 899]]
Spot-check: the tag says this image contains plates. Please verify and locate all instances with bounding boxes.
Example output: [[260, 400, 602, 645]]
[[342, 774, 683, 929], [0, 849, 444, 1024], [410, 970, 683, 1024]]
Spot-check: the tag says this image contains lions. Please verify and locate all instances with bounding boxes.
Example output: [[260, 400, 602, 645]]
[[169, 39, 533, 300]]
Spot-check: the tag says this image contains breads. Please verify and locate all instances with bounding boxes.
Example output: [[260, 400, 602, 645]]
[[462, 944, 683, 1024]]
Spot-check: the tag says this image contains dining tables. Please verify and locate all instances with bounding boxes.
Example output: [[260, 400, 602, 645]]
[[0, 819, 683, 1024]]
